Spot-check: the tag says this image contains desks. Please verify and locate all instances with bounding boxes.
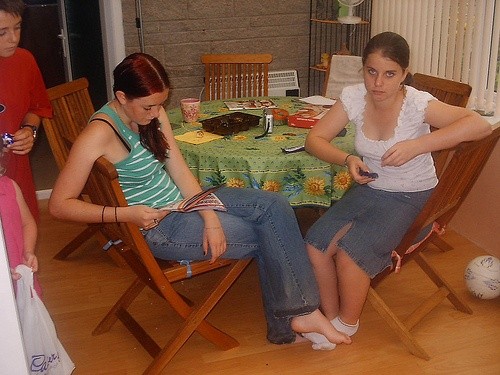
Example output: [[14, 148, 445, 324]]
[[166, 96, 362, 209]]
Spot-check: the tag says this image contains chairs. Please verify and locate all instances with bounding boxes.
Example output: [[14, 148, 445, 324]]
[[41, 78, 253, 375], [201, 53, 274, 101], [366, 73, 499, 361]]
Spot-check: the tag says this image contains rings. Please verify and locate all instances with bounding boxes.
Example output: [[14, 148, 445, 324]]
[[154, 219, 159, 223]]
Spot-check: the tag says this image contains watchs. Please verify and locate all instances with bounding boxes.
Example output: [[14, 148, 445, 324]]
[[20, 124, 37, 140]]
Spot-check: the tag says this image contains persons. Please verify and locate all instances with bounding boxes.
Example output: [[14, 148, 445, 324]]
[[48, 53, 353, 344], [0, 176, 43, 300], [300, 31, 492, 350], [0, 0, 53, 222]]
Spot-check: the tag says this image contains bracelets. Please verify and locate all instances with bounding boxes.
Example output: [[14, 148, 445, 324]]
[[114, 206, 117, 222], [203, 225, 223, 230], [343, 154, 352, 165], [101, 204, 107, 223]]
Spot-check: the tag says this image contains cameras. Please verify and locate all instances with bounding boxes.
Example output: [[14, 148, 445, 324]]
[[263, 107, 273, 133], [0, 133, 14, 149]]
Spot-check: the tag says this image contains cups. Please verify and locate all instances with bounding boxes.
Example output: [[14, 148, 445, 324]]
[[180, 98, 201, 123]]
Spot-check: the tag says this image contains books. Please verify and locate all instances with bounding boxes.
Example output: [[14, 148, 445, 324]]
[[161, 184, 227, 213]]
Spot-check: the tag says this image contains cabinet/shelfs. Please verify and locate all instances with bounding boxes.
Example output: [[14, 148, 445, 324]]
[[309, 18, 370, 72]]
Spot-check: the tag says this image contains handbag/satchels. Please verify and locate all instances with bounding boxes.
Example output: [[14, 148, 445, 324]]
[[13, 264, 75, 375]]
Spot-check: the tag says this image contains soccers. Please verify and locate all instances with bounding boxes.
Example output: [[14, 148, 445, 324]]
[[465, 256, 500, 300]]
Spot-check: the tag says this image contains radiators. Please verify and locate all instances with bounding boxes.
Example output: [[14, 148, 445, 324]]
[[210, 70, 301, 101]]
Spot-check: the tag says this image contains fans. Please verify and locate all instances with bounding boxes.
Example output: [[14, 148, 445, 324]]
[[337, 0, 365, 25]]
